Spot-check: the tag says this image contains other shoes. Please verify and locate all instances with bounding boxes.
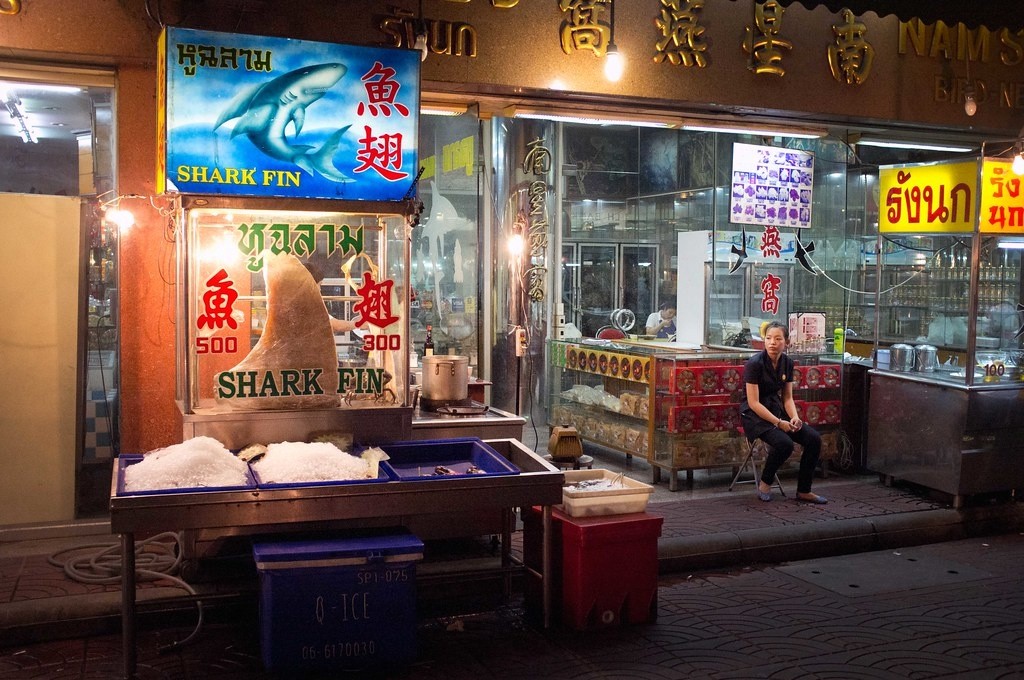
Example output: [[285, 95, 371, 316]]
[[759, 488, 772, 502], [797, 493, 829, 503]]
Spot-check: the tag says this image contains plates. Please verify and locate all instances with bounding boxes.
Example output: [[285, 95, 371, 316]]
[[948, 371, 983, 378]]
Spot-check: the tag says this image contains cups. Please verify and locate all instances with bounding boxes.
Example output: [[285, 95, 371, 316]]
[[791, 338, 827, 358]]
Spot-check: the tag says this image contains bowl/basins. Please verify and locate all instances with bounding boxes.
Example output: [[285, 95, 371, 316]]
[[976, 348, 1024, 373]]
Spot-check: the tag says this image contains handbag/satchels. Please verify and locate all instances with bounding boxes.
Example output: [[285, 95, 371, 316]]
[[928, 314, 955, 345], [952, 317, 967, 346]]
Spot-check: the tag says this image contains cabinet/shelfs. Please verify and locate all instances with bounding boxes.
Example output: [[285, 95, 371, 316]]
[[648, 353, 842, 491], [547, 338, 697, 483]]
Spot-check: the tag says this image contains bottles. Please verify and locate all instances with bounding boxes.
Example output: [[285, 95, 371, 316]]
[[834, 323, 845, 355], [424, 325, 434, 356], [943, 355, 958, 366]]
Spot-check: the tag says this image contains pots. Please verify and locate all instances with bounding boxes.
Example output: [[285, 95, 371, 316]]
[[436, 405, 488, 419], [439, 312, 477, 340], [422, 354, 468, 400]]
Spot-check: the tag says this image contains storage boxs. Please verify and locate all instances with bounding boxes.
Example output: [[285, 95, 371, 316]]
[[242, 442, 391, 488], [668, 366, 842, 466], [248, 526, 426, 679], [521, 504, 664, 634], [550, 340, 651, 385], [365, 437, 520, 481], [116, 448, 258, 496], [552, 468, 656, 518]]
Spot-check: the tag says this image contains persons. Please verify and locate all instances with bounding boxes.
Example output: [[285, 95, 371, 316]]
[[738, 321, 827, 504], [915, 300, 1019, 345], [645, 301, 676, 337]]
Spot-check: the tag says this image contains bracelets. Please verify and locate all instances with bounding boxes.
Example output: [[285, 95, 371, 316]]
[[774, 418, 782, 430]]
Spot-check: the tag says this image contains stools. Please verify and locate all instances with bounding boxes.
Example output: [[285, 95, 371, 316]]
[[729, 424, 786, 500]]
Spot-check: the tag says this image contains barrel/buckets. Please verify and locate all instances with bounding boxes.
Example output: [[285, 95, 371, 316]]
[[889, 344, 916, 372], [914, 344, 938, 373]]
